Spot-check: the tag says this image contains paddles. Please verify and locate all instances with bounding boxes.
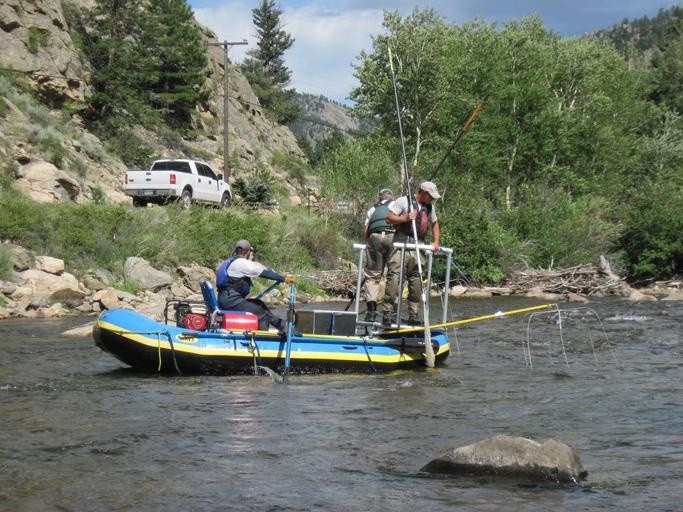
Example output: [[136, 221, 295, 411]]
[[281, 277, 296, 384]]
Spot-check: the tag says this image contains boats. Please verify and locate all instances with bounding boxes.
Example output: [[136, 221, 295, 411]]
[[92, 307, 450, 377]]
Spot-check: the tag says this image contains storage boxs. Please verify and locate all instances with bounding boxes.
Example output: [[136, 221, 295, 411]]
[[295, 310, 357, 336]]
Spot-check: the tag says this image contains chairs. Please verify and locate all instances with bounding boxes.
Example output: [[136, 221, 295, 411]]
[[199, 281, 258, 332]]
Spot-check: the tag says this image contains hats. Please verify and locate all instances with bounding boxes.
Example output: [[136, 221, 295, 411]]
[[379, 189, 393, 196], [420, 182, 442, 199], [236, 239, 254, 250]]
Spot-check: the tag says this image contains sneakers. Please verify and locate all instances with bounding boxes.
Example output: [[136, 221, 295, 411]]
[[383, 313, 396, 324], [281, 311, 296, 335], [365, 311, 376, 322], [409, 316, 423, 326]]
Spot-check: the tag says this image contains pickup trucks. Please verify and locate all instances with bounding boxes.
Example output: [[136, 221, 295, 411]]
[[122, 159, 232, 210]]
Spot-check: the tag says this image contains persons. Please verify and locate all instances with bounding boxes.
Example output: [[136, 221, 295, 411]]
[[364, 189, 397, 322], [217, 240, 297, 335], [382, 182, 442, 324]]
